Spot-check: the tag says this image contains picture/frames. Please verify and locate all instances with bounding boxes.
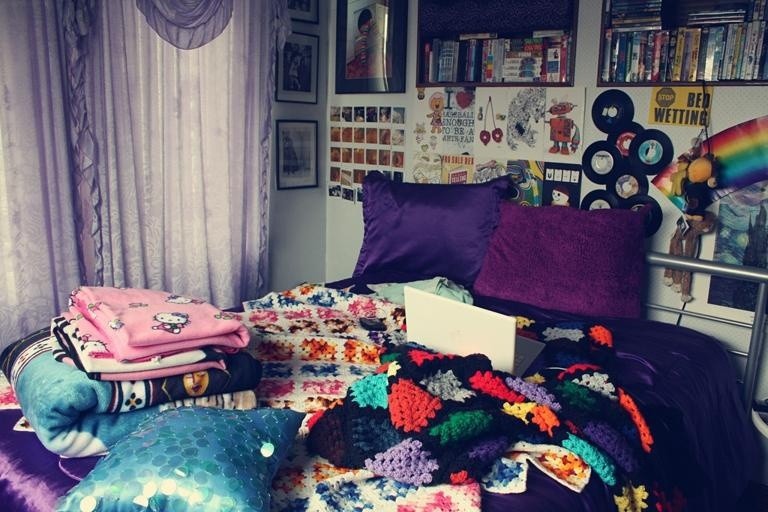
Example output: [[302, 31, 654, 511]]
[[279, 0, 319, 25], [274, 117, 320, 190], [276, 31, 320, 106], [336, 0, 407, 96]]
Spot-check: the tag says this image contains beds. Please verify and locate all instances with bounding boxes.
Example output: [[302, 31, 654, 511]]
[[1, 238, 768, 512]]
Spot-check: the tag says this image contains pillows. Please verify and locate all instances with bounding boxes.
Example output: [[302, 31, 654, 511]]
[[478, 196, 653, 322], [50, 407, 306, 512], [352, 165, 516, 285]]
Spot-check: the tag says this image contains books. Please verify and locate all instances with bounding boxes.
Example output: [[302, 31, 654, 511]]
[[424, 25, 572, 83], [602, 1, 763, 82]]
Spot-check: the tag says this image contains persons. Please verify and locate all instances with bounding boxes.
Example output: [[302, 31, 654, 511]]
[[426, 92, 444, 134]]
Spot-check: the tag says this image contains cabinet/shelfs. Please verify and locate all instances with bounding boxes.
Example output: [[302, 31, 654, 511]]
[[419, 5, 574, 86], [597, 0, 768, 87]]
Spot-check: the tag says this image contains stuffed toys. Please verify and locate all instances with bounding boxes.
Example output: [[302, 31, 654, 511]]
[[663, 153, 718, 302]]
